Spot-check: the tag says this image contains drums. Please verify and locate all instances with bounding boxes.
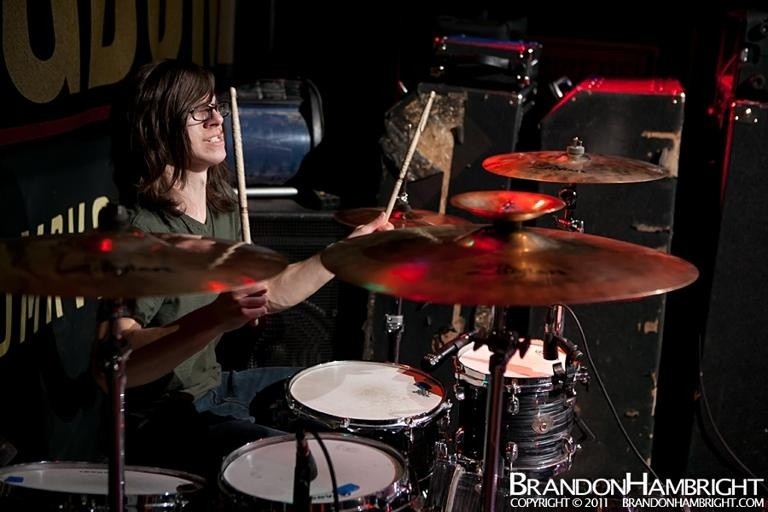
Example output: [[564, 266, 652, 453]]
[[420, 441, 692, 512], [283, 359, 452, 509], [453, 337, 590, 481], [0, 460, 208, 512], [218, 433, 411, 512]]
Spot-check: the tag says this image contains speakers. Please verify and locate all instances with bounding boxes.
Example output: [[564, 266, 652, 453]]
[[216, 209, 388, 371]]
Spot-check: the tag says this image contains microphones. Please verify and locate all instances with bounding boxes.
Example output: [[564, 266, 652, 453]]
[[418, 330, 481, 372], [543, 306, 558, 361], [294, 430, 318, 482]]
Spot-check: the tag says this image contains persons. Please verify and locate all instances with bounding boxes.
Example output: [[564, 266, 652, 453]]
[[93, 59, 394, 479]]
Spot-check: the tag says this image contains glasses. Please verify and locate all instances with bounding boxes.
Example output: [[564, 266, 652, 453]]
[[188, 101, 231, 121]]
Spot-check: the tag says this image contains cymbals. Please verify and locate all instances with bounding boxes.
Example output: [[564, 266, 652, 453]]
[[0, 231, 287, 296], [321, 226, 700, 305], [481, 150, 670, 185], [451, 191, 565, 221], [335, 206, 473, 228]]
